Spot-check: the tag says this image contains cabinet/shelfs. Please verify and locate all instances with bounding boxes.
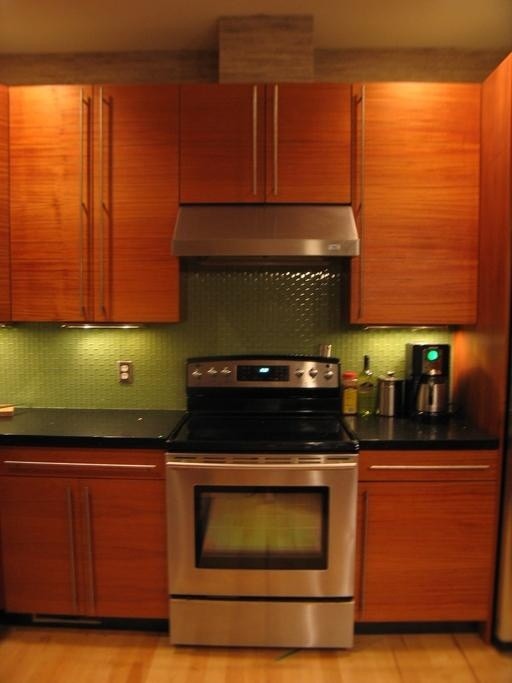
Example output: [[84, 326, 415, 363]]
[[183, 85, 352, 206], [0, 83, 182, 323], [355, 449, 499, 624], [352, 84, 478, 324], [0, 446, 169, 620]]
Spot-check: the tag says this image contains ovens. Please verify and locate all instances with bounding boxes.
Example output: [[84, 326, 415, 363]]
[[167, 460, 359, 600]]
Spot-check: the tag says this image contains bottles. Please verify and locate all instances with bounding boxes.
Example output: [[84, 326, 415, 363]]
[[358, 355, 373, 415], [343, 370, 358, 414], [378, 371, 404, 416]]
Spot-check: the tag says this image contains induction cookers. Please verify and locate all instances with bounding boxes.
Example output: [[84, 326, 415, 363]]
[[167, 411, 358, 449]]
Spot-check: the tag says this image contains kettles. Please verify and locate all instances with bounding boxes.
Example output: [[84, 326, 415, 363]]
[[417, 374, 447, 418]]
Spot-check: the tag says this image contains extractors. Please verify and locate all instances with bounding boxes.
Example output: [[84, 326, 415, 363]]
[[170, 202, 361, 267]]
